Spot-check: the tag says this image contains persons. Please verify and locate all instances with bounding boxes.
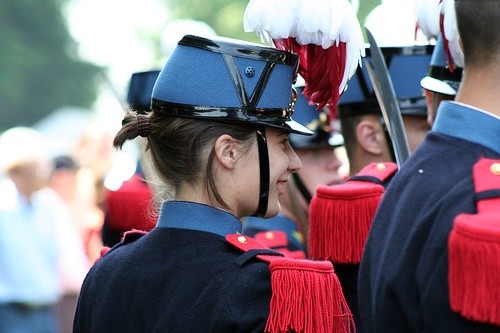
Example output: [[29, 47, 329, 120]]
[[243, 85, 343, 259], [1, 118, 104, 333], [356, 0, 500, 333], [306, 46, 437, 321], [73, 33, 359, 333]]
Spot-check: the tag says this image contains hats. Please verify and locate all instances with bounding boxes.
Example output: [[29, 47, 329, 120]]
[[338, 44, 425, 116], [128, 35, 346, 148], [420, 35, 462, 98]]
